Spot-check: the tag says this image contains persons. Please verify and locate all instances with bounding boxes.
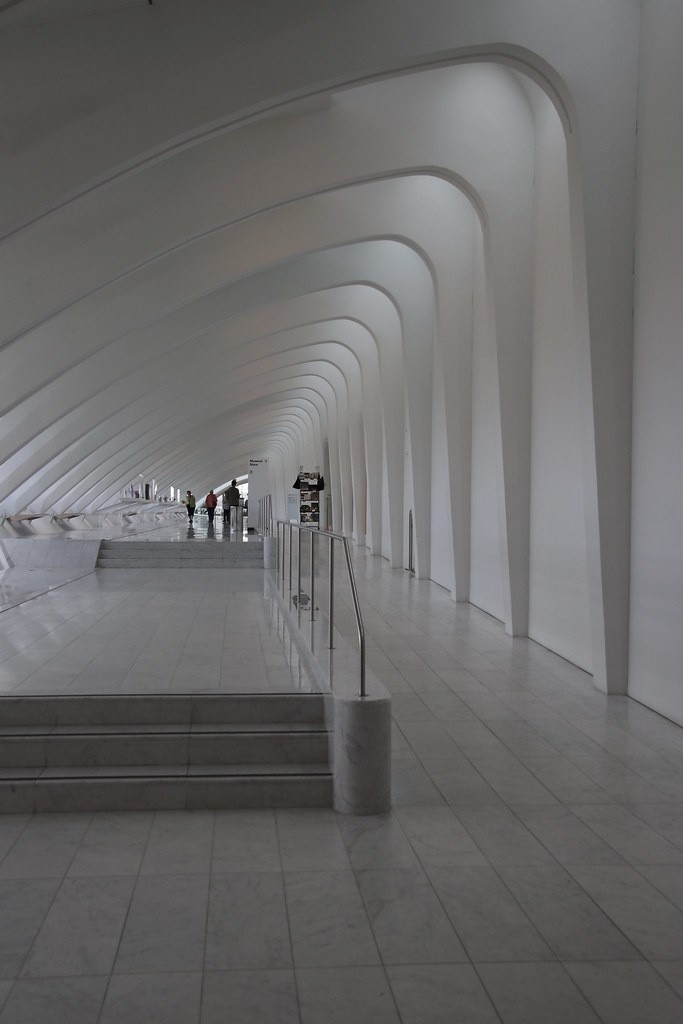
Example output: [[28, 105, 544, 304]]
[[221, 491, 229, 524], [205, 489, 217, 523], [226, 479, 240, 527], [185, 491, 196, 522]]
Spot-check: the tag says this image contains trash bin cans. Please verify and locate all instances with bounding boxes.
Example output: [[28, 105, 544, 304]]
[[230, 506, 243, 532]]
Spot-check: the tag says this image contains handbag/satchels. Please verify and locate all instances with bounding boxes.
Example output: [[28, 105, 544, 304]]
[[186, 504, 191, 510]]
